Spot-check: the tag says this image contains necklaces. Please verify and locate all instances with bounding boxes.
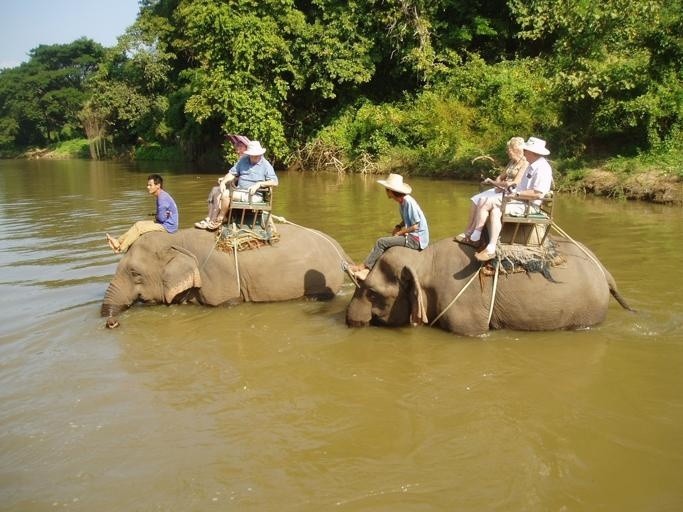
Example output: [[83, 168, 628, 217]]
[[507, 156, 523, 175]]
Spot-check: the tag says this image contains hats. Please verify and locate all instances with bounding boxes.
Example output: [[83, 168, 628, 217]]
[[243, 141, 266, 156], [520, 137, 551, 156], [507, 137, 525, 162], [376, 173, 413, 195]]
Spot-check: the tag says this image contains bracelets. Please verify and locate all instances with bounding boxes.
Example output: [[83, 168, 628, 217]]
[[515, 192, 519, 201], [395, 225, 401, 229]]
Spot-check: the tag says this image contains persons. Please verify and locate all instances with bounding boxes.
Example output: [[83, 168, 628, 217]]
[[452, 135, 529, 248], [203, 140, 279, 230], [340, 172, 430, 290], [473, 136, 553, 262], [105, 174, 178, 255], [193, 133, 251, 229]]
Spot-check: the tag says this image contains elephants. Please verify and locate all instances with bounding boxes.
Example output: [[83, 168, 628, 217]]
[[101, 223, 357, 329], [346, 239, 637, 336]]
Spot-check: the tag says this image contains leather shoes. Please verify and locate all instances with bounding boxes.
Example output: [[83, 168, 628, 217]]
[[475, 250, 497, 262], [195, 216, 224, 229], [456, 228, 480, 247]]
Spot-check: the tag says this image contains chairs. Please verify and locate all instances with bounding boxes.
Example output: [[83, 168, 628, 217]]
[[226, 181, 272, 232], [502, 177, 555, 248]]
